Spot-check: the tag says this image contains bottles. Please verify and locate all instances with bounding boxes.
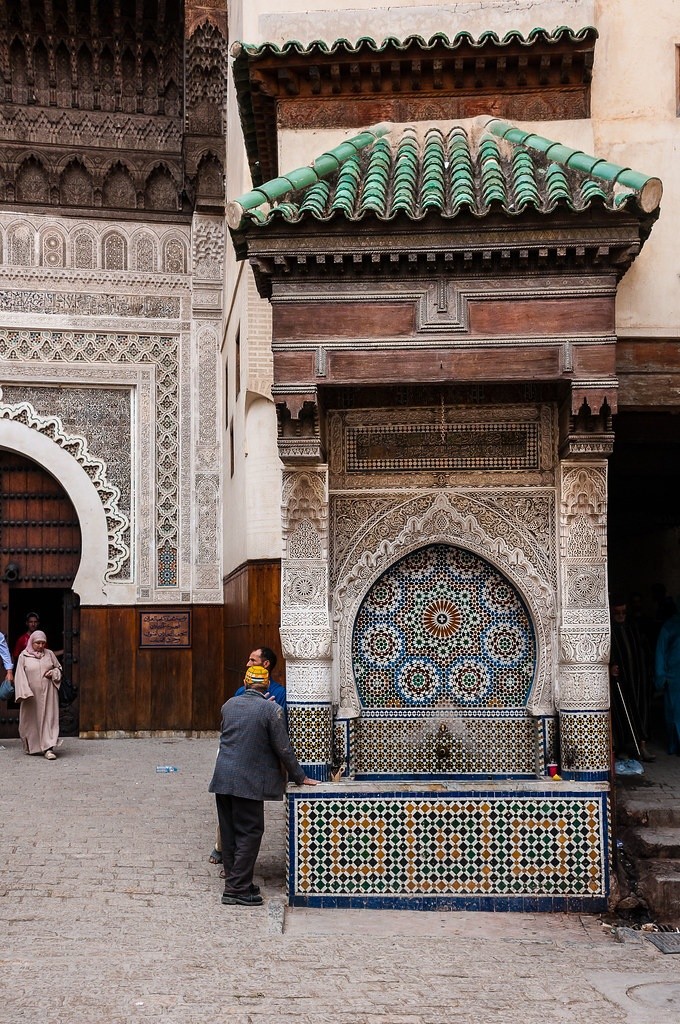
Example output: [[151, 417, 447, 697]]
[[550, 759, 554, 764], [156, 765, 177, 773]]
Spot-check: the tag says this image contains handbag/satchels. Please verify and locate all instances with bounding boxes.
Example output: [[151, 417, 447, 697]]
[[57, 668, 79, 709]]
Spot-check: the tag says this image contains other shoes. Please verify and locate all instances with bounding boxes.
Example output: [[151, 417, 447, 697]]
[[44, 749, 56, 760]]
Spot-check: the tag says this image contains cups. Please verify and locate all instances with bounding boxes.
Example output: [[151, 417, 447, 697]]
[[547, 764, 558, 778]]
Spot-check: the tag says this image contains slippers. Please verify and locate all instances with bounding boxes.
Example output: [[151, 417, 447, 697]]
[[208, 848, 224, 864]]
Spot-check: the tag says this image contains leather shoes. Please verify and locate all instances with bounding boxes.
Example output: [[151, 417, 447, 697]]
[[225, 883, 261, 894], [221, 891, 264, 905]]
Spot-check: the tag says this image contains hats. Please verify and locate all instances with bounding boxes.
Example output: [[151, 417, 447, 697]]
[[245, 666, 270, 684]]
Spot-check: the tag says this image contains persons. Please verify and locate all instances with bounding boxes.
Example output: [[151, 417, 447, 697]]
[[11, 611, 63, 671], [0, 632, 14, 681], [207, 646, 286, 878], [14, 629, 63, 760], [610, 596, 657, 761], [208, 666, 321, 907], [654, 613, 680, 756]]
